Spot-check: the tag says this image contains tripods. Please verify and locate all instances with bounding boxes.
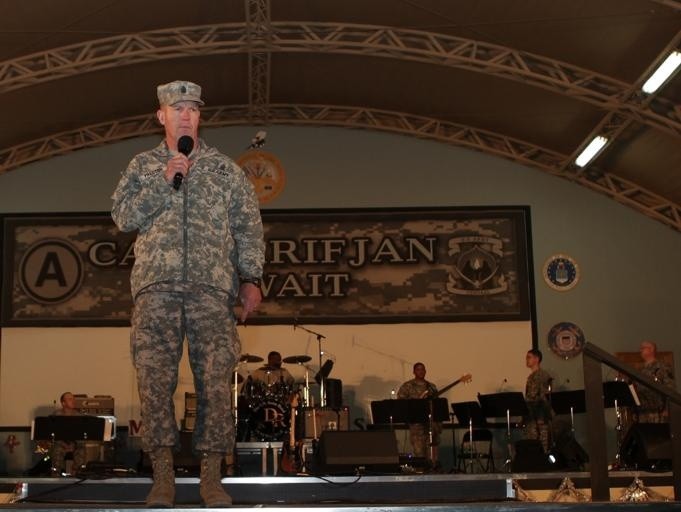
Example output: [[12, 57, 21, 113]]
[[459, 416, 488, 474]]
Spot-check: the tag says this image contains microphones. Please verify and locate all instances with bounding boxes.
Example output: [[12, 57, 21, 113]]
[[293, 317, 298, 331], [172, 136, 195, 191]]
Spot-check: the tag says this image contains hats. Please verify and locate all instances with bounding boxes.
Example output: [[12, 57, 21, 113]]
[[156, 80, 204, 106]]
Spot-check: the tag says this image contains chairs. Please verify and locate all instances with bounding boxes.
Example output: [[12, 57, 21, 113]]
[[456, 428, 495, 474]]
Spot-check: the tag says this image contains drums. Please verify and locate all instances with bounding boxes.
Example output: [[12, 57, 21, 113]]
[[249, 399, 289, 438]]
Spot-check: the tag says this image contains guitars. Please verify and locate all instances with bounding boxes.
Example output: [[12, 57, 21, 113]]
[[421, 375, 472, 398], [280, 395, 303, 475]]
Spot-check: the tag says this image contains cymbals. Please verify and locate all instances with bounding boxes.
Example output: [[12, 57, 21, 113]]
[[295, 380, 318, 385], [283, 356, 312, 363], [232, 371, 243, 384], [239, 353, 263, 362]]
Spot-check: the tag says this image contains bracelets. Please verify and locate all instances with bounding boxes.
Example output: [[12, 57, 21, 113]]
[[240, 278, 261, 288]]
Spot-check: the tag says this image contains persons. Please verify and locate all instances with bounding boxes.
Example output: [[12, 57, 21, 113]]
[[110, 80, 268, 507], [627, 340, 673, 425], [49, 391, 89, 476], [396, 362, 443, 473], [520, 347, 554, 460], [247, 352, 295, 387]]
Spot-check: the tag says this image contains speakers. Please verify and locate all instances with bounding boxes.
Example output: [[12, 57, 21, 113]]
[[619, 421, 675, 471], [310, 430, 399, 474], [139, 431, 227, 473]]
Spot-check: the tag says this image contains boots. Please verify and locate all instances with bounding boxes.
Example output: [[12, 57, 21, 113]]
[[145, 448, 174, 509], [199, 454, 232, 508]]
